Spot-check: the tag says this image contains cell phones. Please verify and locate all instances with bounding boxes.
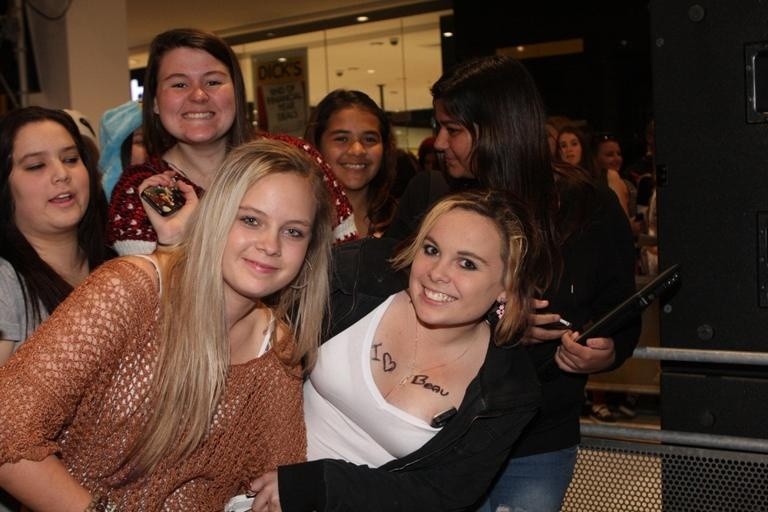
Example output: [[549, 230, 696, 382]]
[[141, 173, 187, 215]]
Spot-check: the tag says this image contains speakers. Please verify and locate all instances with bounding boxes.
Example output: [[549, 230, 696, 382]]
[[648, 0, 768, 376], [660, 373, 768, 512]]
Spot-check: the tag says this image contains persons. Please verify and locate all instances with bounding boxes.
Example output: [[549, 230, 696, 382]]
[[1, 140, 341, 511], [544, 118, 660, 276], [381, 53, 642, 511], [1, 107, 117, 366], [303, 90, 412, 343], [390, 136, 440, 199], [247, 193, 541, 509], [64, 102, 148, 204], [109, 28, 363, 256]]
